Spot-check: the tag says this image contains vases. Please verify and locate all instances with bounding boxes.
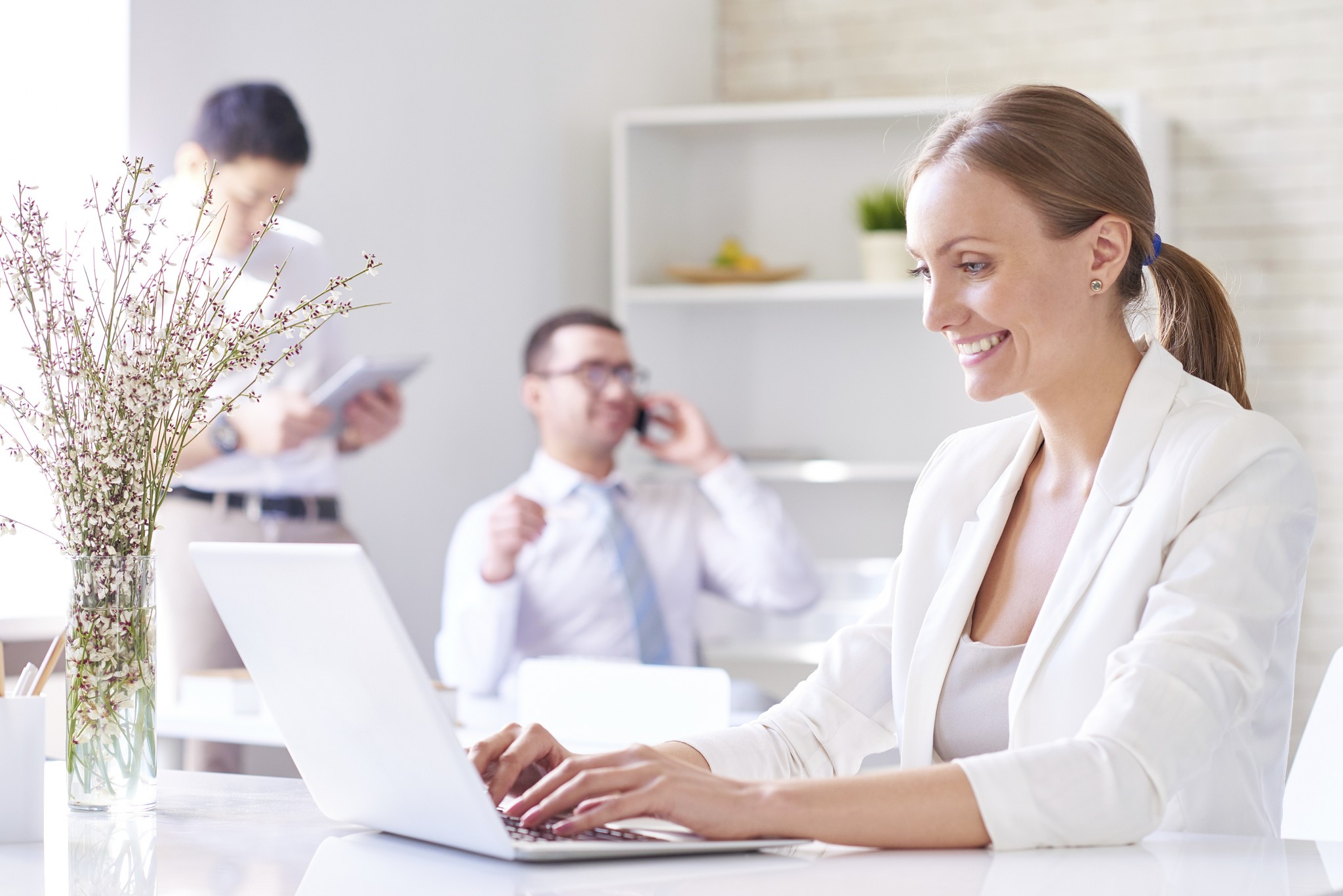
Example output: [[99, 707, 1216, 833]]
[[66, 554, 160, 813]]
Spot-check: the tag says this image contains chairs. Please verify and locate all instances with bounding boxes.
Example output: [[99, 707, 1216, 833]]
[[1283, 646, 1343, 843]]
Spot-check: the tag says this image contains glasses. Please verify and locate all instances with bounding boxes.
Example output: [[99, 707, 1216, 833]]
[[546, 364, 635, 388]]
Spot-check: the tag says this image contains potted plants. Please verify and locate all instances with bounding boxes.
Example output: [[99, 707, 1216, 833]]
[[855, 182, 913, 284]]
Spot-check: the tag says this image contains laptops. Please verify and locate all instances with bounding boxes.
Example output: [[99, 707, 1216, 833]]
[[185, 538, 812, 864]]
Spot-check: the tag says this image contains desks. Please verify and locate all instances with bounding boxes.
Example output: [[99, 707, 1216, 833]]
[[0, 762, 1343, 895], [148, 667, 772, 776]]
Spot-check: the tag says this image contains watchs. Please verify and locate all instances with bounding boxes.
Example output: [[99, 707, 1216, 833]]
[[209, 415, 240, 454]]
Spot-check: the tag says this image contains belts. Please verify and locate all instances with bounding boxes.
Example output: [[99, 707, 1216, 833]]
[[161, 486, 337, 520]]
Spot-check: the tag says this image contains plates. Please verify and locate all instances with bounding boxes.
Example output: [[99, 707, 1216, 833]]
[[669, 266, 803, 284]]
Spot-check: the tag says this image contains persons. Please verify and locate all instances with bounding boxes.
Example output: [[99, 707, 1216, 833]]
[[432, 310, 825, 715], [106, 83, 410, 776], [463, 85, 1322, 852]]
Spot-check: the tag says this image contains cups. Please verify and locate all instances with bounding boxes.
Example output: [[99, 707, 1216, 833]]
[[0, 696, 46, 847]]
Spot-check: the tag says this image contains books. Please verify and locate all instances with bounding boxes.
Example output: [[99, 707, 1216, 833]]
[[307, 355, 426, 438]]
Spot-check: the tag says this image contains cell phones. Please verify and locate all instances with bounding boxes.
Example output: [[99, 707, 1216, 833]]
[[631, 394, 647, 434]]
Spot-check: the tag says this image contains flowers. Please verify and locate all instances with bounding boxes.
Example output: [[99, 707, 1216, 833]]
[[0, 145, 392, 801]]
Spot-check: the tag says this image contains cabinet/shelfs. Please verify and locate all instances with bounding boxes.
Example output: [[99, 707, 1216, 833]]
[[613, 95, 1183, 643]]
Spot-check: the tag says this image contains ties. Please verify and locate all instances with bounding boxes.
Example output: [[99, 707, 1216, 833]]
[[595, 481, 670, 665]]
